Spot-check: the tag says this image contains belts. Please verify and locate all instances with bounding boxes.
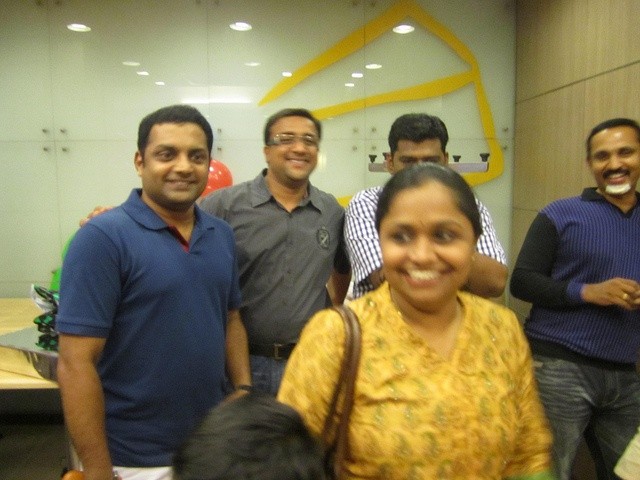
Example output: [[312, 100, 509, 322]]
[[248, 342, 296, 360]]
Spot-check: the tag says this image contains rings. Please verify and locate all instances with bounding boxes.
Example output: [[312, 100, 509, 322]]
[[622, 293, 627, 299]]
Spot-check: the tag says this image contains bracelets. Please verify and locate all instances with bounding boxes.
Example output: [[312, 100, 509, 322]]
[[233, 385, 256, 395]]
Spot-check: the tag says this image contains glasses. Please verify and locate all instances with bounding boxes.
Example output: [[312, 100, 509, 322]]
[[267, 133, 318, 146]]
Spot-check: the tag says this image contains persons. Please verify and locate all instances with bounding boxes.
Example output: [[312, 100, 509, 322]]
[[174, 390, 336, 479], [275, 162, 554, 479], [54, 104, 253, 480], [343, 113, 508, 305], [79, 109, 352, 401], [509, 117, 640, 479]]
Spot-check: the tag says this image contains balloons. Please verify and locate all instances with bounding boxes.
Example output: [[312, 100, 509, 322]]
[[196, 158, 233, 201]]
